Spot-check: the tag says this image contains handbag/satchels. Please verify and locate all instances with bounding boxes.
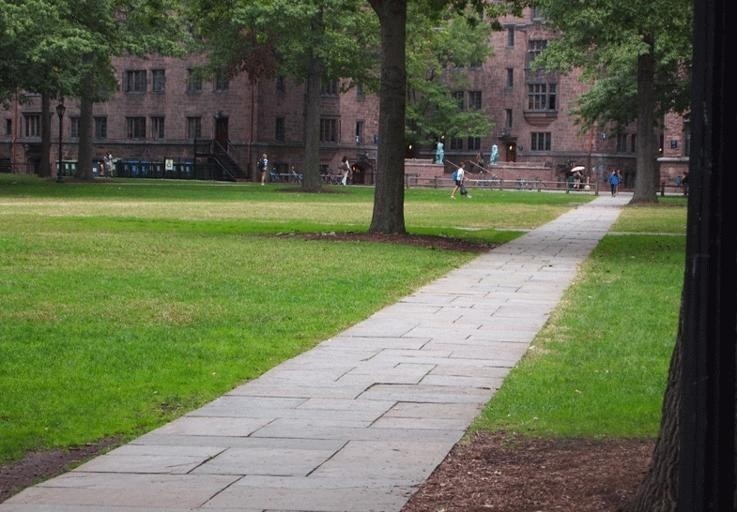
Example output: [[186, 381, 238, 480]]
[[343, 164, 347, 171], [460, 184, 467, 195]]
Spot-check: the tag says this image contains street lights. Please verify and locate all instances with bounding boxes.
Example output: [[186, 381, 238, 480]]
[[54, 103, 67, 183]]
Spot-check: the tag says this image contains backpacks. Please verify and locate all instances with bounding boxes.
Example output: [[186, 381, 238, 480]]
[[259, 158, 265, 171], [452, 170, 458, 181]]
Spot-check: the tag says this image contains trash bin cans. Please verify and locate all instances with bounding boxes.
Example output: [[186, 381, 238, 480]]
[[116, 157, 193, 178], [568, 176, 574, 188], [92, 160, 104, 177], [56, 160, 76, 177], [276, 160, 289, 174]]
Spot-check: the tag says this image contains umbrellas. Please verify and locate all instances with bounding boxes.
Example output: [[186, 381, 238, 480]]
[[571, 166, 585, 172]]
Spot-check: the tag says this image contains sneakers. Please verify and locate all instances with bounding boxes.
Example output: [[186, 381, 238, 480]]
[[449, 196, 456, 200], [467, 195, 472, 198]]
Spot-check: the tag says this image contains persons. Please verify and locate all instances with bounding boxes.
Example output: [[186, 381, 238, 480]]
[[568, 171, 585, 190], [451, 162, 472, 200], [681, 171, 689, 196], [98, 151, 113, 176], [608, 169, 622, 197], [476, 151, 485, 173], [258, 153, 268, 186], [339, 156, 351, 186]]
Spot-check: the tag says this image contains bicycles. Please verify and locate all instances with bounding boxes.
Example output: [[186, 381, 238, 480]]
[[268, 164, 343, 186]]
